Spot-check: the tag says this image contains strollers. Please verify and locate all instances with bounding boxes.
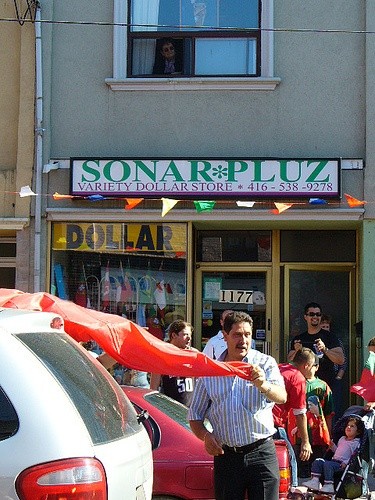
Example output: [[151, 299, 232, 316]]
[[288, 405, 375, 500]]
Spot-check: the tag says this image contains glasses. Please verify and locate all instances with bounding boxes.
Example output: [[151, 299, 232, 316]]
[[306, 312, 321, 317], [161, 46, 175, 52]]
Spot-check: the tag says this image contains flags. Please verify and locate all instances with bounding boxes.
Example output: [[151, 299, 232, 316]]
[[65, 260, 187, 331]]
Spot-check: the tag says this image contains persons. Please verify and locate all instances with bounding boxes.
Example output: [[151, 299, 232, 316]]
[[187, 311, 287, 500], [74, 302, 375, 500], [148, 36, 186, 75]]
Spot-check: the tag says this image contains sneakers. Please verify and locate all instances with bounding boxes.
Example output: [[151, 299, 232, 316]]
[[319, 483, 334, 493], [302, 479, 319, 490]]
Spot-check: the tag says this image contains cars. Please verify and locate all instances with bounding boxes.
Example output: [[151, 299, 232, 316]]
[[120, 385, 292, 500]]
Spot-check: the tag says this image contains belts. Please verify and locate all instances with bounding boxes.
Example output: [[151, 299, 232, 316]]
[[222, 435, 273, 454]]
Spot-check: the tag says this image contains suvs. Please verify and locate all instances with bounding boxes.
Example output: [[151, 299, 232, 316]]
[[0, 308, 154, 500]]
